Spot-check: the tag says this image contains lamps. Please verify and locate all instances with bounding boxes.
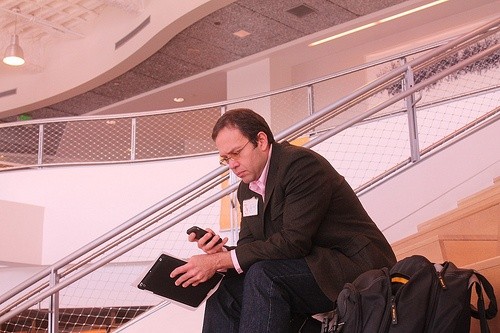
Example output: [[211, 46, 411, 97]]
[[2, 5, 27, 69]]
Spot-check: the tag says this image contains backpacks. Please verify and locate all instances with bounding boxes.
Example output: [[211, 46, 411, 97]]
[[318, 254, 500, 332]]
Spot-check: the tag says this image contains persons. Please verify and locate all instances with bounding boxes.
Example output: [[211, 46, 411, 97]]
[[171, 108, 397, 333]]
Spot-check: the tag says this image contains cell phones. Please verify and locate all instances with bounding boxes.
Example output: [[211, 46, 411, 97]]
[[187, 226, 222, 247]]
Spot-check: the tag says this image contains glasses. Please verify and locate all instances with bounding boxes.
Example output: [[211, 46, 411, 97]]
[[219, 137, 257, 166]]
